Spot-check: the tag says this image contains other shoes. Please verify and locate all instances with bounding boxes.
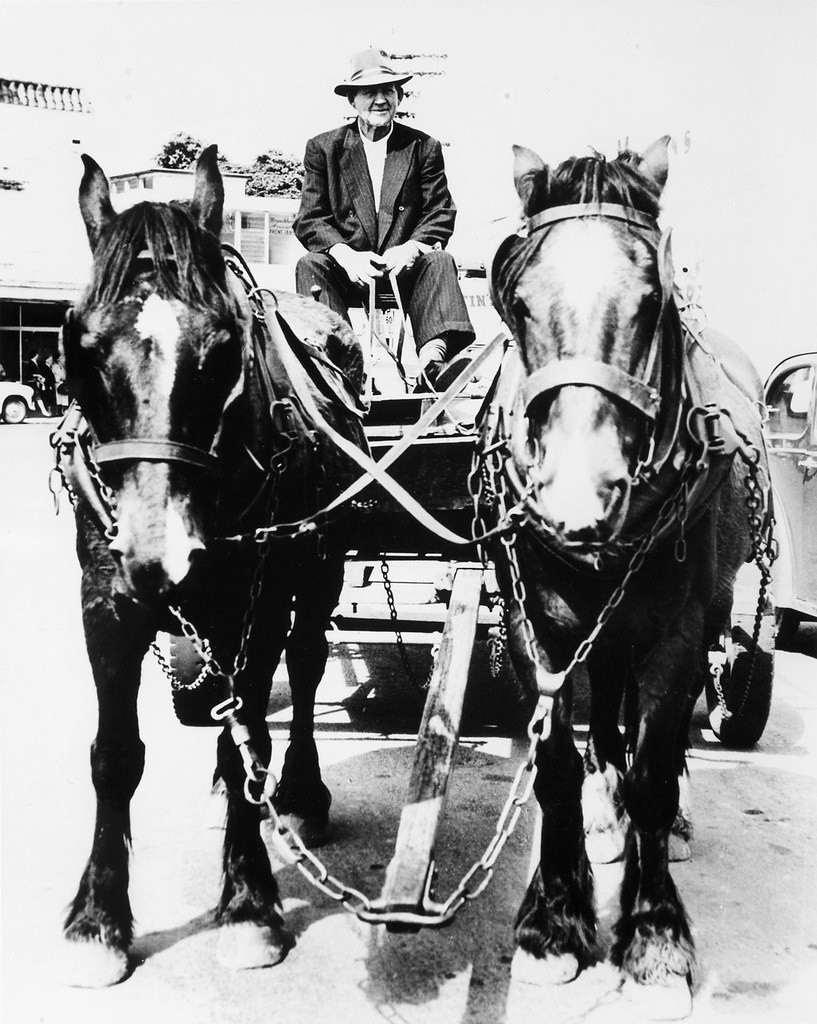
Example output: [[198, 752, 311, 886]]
[[52, 412, 65, 417], [414, 353, 472, 394], [41, 413, 52, 418]]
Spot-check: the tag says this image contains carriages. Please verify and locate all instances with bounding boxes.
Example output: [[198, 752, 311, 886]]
[[55, 130, 778, 1024]]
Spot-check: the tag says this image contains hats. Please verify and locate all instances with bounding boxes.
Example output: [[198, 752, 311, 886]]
[[333, 49, 413, 97], [44, 353, 52, 360], [29, 350, 39, 359]]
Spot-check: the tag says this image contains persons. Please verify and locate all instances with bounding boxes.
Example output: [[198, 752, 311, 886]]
[[39, 353, 56, 416], [25, 352, 51, 418], [53, 353, 69, 416], [292, 48, 477, 394]]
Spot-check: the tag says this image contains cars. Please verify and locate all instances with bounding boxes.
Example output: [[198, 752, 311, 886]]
[[0, 362, 40, 423], [753, 354, 817, 647]]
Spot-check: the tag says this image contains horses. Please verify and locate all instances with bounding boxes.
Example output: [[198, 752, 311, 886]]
[[479, 133, 780, 1021], [42, 141, 375, 995]]
